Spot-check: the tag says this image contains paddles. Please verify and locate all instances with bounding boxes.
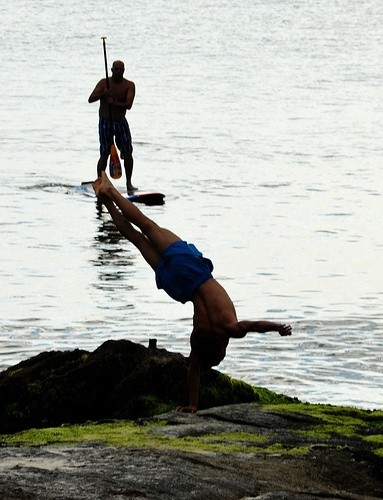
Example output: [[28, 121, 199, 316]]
[[99, 35, 125, 180]]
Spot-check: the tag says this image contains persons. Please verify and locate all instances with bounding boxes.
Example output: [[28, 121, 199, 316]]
[[89, 61, 137, 195], [93, 170, 292, 413]]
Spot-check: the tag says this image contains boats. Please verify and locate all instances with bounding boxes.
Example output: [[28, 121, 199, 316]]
[[25, 184, 164, 202]]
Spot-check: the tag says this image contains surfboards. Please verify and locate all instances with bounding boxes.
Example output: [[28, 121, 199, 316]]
[[78, 183, 163, 205]]
